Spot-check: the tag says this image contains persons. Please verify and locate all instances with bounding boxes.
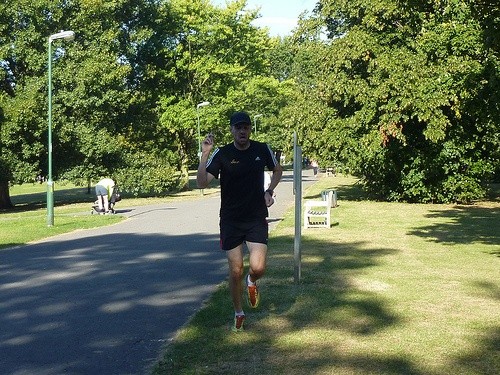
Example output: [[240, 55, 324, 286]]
[[197, 113, 282, 335], [94, 177, 117, 217], [311, 159, 318, 175], [90, 192, 122, 216]]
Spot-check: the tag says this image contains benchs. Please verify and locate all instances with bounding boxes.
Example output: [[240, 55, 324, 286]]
[[303, 190, 333, 228]]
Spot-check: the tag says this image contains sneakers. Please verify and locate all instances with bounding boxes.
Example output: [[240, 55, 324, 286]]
[[246, 275, 260, 308], [235, 311, 245, 330]]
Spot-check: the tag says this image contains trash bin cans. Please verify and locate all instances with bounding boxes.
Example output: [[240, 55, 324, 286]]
[[321, 188, 337, 208]]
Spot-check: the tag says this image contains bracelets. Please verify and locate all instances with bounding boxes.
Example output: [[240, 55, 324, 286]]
[[265, 187, 274, 196]]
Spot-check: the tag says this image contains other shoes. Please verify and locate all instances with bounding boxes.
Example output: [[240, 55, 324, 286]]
[[98, 209, 112, 215]]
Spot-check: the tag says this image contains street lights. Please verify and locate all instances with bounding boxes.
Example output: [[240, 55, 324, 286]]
[[197, 101, 210, 196], [46, 31, 75, 225], [254, 114, 262, 134]]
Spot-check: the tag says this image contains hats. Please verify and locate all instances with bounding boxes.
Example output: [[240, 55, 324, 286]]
[[230, 112, 252, 126]]
